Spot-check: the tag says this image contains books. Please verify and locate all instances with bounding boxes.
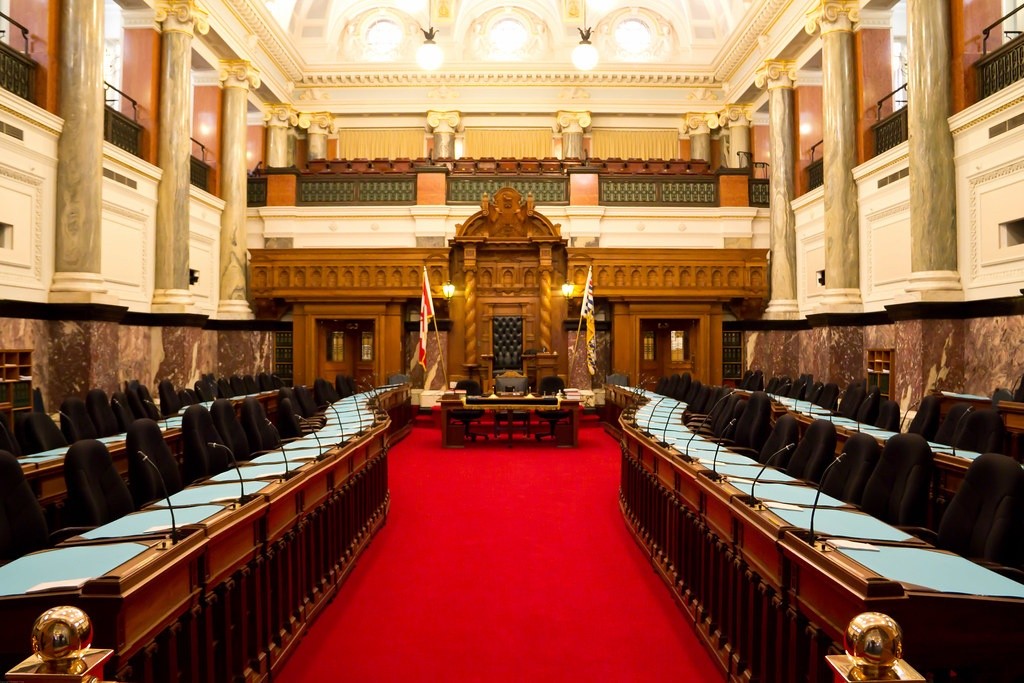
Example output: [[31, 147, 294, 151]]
[[563, 388, 580, 399], [442, 390, 467, 400]]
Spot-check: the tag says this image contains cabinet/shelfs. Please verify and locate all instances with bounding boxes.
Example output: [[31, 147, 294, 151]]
[[865, 347, 898, 400], [0, 347, 36, 418]]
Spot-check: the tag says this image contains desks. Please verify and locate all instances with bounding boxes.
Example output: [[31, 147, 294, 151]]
[[437, 393, 585, 445], [0, 383, 415, 683], [599, 385, 1023, 682]]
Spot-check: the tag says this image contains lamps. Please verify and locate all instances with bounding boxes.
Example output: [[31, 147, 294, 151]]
[[443, 279, 456, 302], [571, 1, 602, 70], [414, 1, 442, 74], [563, 281, 574, 300]]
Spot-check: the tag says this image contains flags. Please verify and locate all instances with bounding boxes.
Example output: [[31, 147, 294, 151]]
[[580, 265, 597, 375], [418, 272, 435, 372]]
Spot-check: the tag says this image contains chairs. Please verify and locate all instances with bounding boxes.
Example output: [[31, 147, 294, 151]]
[[655, 370, 1024, 583], [0, 372, 409, 560], [535, 376, 567, 442], [451, 380, 488, 441], [492, 376, 533, 438]]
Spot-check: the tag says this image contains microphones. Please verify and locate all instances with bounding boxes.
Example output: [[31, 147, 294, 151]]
[[952, 406, 974, 456], [55, 369, 404, 545], [900, 401, 919, 434], [630, 370, 874, 545]]
